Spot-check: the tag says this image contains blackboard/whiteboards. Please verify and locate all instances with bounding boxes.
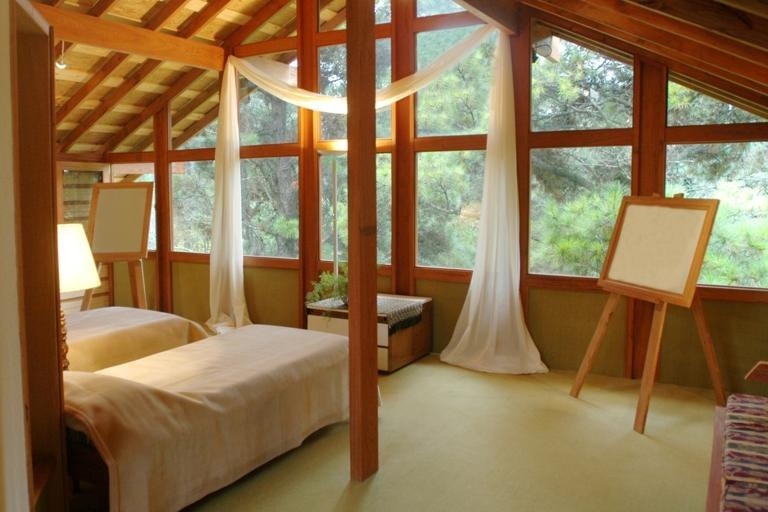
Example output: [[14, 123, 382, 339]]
[[87, 181, 153, 262], [597, 195, 720, 308]]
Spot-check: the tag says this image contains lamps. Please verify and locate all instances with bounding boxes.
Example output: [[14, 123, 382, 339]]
[[56, 223, 103, 371], [317, 149, 347, 299]]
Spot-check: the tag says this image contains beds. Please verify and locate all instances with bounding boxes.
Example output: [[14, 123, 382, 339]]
[[60, 323, 383, 512], [63, 306, 209, 372]]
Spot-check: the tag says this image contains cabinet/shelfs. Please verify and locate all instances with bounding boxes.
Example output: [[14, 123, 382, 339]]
[[306, 292, 434, 374]]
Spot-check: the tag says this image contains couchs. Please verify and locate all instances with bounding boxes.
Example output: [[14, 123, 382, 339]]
[[704, 361, 768, 512]]
